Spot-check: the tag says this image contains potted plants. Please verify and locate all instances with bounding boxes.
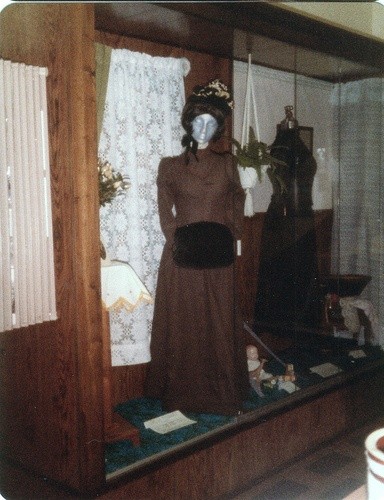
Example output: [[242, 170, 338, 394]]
[[235, 49, 297, 194], [98, 153, 135, 267]]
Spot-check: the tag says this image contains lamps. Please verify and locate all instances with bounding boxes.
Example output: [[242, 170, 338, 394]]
[[101, 260, 155, 446]]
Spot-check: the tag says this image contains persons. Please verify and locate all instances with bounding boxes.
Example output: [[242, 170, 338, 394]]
[[257, 105, 324, 360], [141, 78, 251, 419]]
